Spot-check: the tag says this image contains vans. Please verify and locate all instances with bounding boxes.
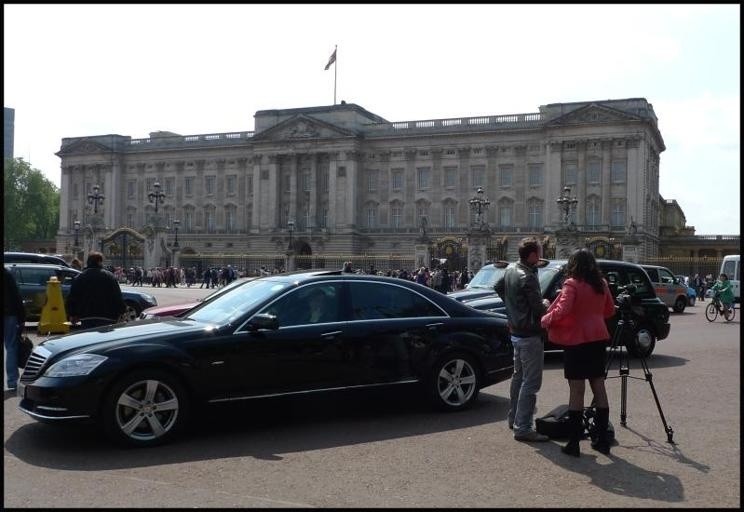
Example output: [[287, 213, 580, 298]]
[[717, 254, 740, 300], [2, 252, 152, 327], [444, 259, 697, 358]]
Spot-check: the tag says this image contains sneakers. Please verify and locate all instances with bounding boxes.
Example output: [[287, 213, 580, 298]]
[[515, 432, 548, 442]]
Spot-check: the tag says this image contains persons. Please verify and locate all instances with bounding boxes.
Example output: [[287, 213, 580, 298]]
[[493, 240, 552, 443], [357, 262, 474, 295], [67, 251, 127, 330], [691, 271, 735, 319], [2, 268, 26, 392], [541, 248, 619, 456], [103, 264, 287, 289]]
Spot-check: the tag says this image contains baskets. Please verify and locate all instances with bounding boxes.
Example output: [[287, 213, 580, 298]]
[[705, 290, 715, 298]]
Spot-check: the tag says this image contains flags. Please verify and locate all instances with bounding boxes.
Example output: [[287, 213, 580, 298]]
[[324, 48, 336, 70]]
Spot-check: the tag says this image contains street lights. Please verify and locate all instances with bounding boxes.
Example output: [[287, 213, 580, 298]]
[[148, 184, 164, 218], [74, 219, 81, 246], [172, 219, 180, 249], [88, 185, 104, 214], [286, 221, 296, 247], [555, 186, 578, 226], [468, 186, 490, 226]]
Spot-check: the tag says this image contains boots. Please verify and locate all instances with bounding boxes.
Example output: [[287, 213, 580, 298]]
[[561, 410, 582, 457], [590, 408, 611, 455]]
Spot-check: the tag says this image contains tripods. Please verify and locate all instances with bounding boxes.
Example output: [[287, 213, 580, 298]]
[[585, 315, 674, 443]]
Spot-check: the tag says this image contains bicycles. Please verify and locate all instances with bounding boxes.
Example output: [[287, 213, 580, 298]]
[[706, 295, 736, 323]]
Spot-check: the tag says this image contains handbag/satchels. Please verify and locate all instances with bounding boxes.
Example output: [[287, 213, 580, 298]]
[[547, 316, 585, 346], [18, 337, 32, 368]]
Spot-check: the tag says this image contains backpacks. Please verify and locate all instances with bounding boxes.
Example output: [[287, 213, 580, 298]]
[[536, 404, 585, 440]]
